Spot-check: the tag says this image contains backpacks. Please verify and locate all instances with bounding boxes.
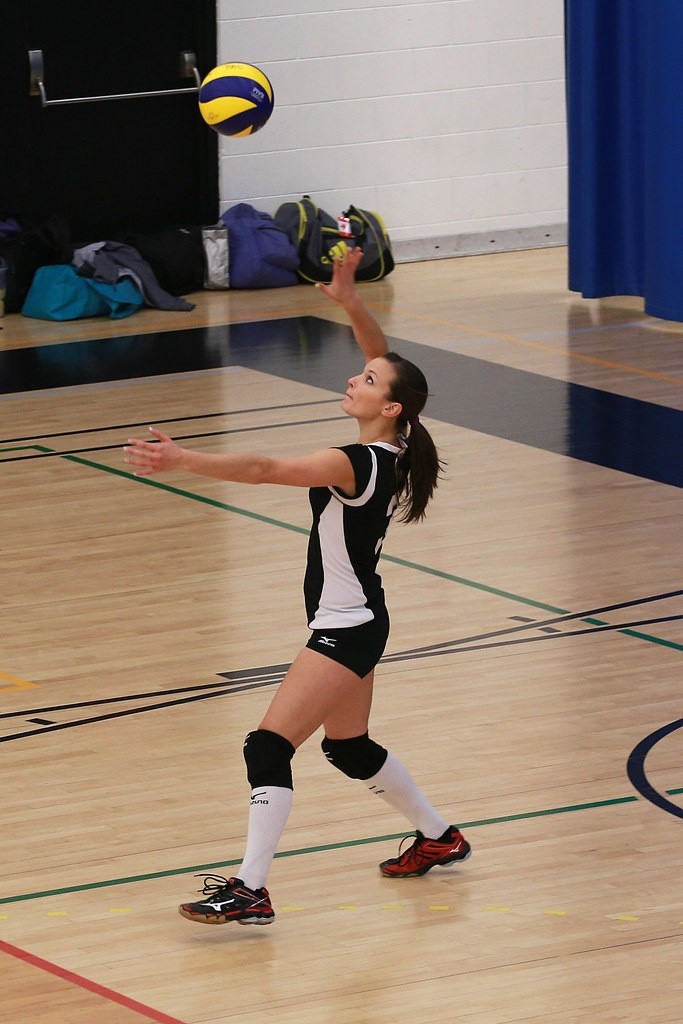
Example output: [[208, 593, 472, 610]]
[[219, 202, 300, 288]]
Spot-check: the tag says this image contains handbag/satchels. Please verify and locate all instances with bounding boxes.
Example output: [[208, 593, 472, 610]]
[[275, 195, 396, 286], [198, 218, 231, 292], [22, 264, 143, 321]]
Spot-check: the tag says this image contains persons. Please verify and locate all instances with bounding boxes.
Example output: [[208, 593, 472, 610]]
[[123, 247, 473, 925]]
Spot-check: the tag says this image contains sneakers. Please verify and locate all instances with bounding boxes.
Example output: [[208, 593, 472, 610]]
[[178, 873, 276, 925], [379, 823, 472, 878]]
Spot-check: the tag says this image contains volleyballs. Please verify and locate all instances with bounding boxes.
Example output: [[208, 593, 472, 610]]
[[197, 61, 276, 140]]
